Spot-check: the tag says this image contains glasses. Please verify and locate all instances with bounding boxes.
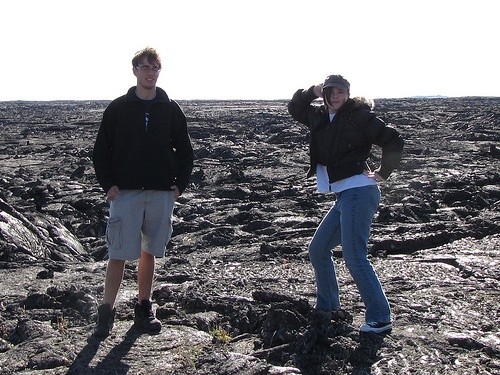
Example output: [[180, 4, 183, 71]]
[[136, 66, 161, 73]]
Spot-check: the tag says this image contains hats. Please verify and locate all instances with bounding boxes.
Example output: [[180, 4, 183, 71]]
[[323, 75, 350, 90]]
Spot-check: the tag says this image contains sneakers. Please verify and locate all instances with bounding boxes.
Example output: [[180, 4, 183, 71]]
[[135, 299, 161, 330], [94, 303, 116, 335], [360, 318, 393, 333]]
